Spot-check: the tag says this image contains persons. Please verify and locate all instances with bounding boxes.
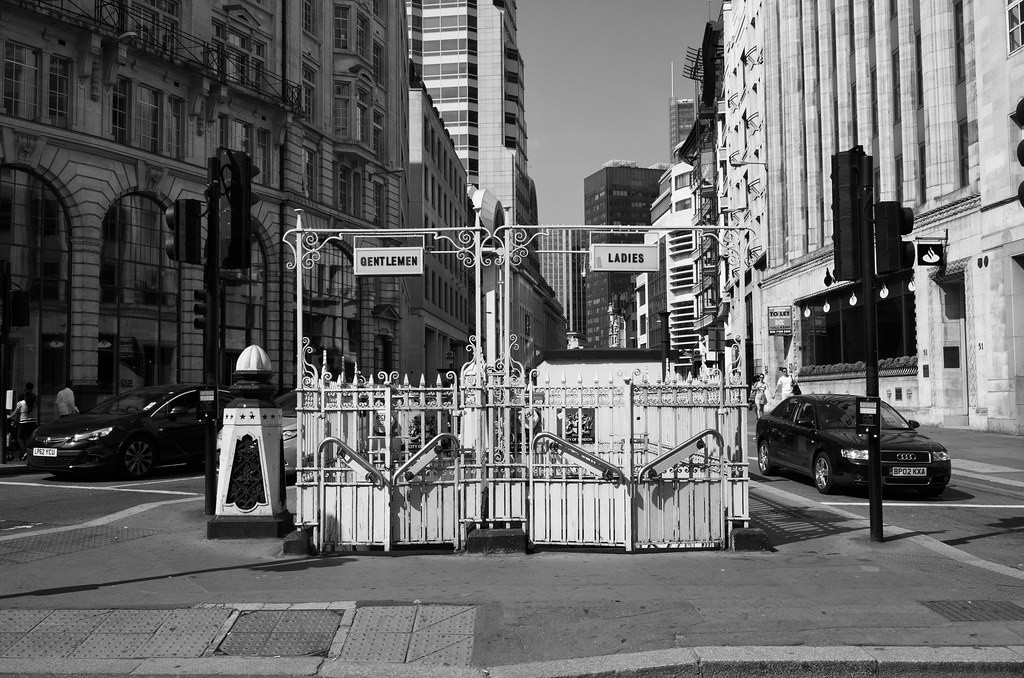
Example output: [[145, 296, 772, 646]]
[[772, 367, 798, 403], [56, 379, 80, 419], [749, 374, 760, 419], [751, 373, 774, 419], [6, 381, 41, 461]]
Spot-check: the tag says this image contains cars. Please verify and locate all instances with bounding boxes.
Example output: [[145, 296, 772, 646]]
[[755, 393, 951, 492], [27, 384, 232, 477], [217, 388, 420, 476]]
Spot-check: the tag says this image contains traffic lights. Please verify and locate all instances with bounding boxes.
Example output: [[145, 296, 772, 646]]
[[829, 147, 873, 280], [218, 146, 260, 267], [875, 200, 917, 274], [165, 199, 200, 265]]
